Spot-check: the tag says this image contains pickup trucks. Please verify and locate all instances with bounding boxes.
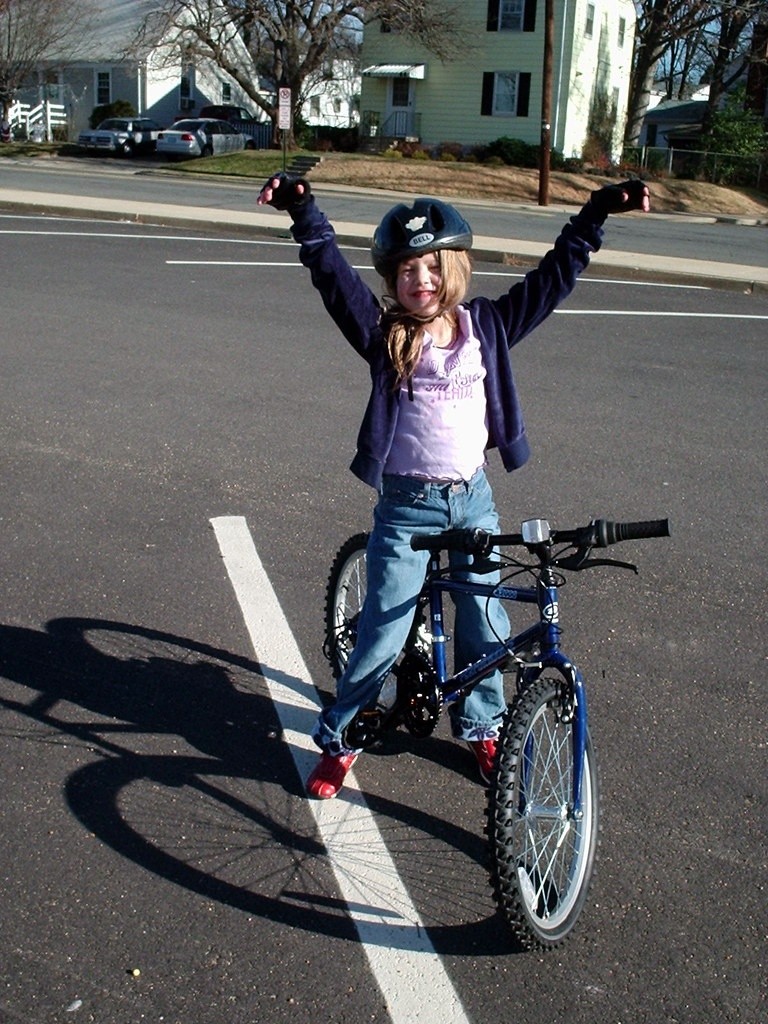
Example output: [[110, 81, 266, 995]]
[[174, 106, 260, 125]]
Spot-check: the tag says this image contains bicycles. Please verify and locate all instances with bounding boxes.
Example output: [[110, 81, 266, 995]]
[[322, 517, 674, 953]]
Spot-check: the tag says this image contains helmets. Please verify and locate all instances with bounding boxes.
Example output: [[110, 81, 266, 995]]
[[372, 198, 474, 278]]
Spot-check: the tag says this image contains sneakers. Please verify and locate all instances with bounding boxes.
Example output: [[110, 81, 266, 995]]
[[466, 739, 498, 785], [305, 750, 359, 800]]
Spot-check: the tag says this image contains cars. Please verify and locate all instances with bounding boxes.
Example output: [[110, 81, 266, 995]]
[[77, 118, 166, 158], [156, 118, 257, 157]]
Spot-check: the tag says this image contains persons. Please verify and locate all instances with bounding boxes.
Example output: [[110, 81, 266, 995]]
[[256, 169, 650, 803]]
